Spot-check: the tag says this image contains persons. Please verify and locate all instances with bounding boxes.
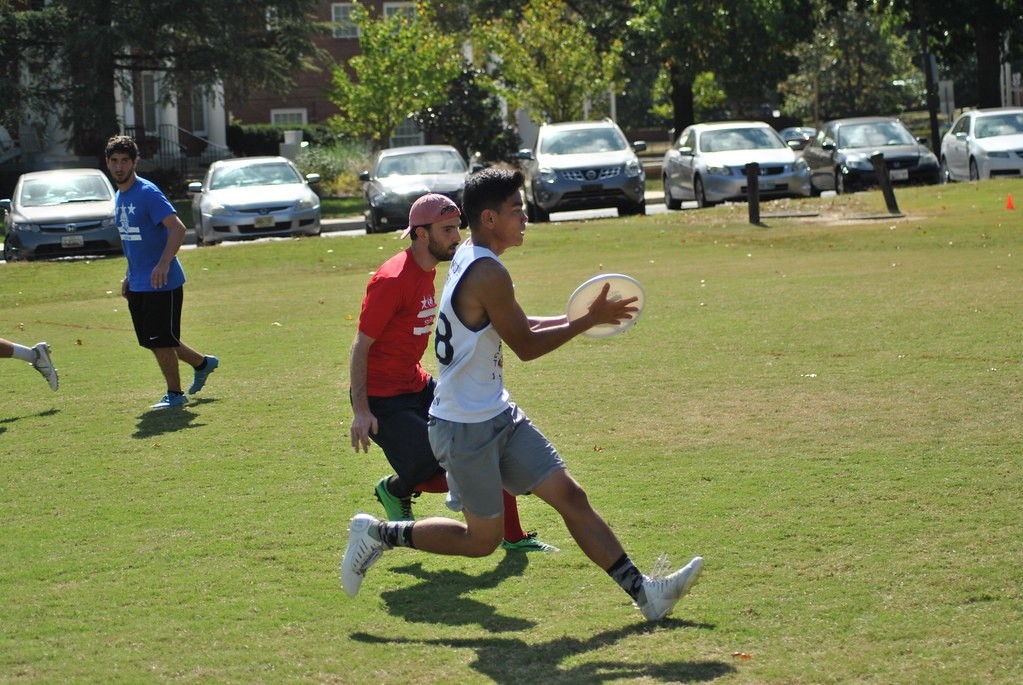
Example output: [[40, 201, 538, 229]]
[[348, 193, 560, 554], [340, 169, 705, 623], [0, 338, 59, 392], [105, 134, 219, 407]]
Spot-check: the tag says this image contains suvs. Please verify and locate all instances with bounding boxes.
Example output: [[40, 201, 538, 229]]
[[517, 116, 647, 223]]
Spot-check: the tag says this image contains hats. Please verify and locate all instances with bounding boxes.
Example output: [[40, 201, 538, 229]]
[[400, 192, 462, 241]]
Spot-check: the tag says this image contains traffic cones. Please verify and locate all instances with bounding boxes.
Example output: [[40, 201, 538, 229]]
[[1006, 194, 1014, 210]]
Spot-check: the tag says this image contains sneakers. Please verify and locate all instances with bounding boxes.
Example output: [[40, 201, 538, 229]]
[[501, 532, 559, 554], [189, 355, 217, 395], [150, 393, 189, 408], [637, 552, 704, 622], [339, 514, 392, 598], [29, 343, 58, 392], [373, 474, 415, 522]]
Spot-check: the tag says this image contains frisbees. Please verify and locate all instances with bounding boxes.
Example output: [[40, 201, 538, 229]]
[[565, 272, 648, 339]]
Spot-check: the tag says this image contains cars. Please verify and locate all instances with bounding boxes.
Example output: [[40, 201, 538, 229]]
[[359, 144, 485, 234], [941, 107, 1023, 184], [804, 117, 939, 197], [661, 120, 811, 209], [188, 156, 323, 247], [0, 168, 123, 263], [779, 126, 835, 151]]
